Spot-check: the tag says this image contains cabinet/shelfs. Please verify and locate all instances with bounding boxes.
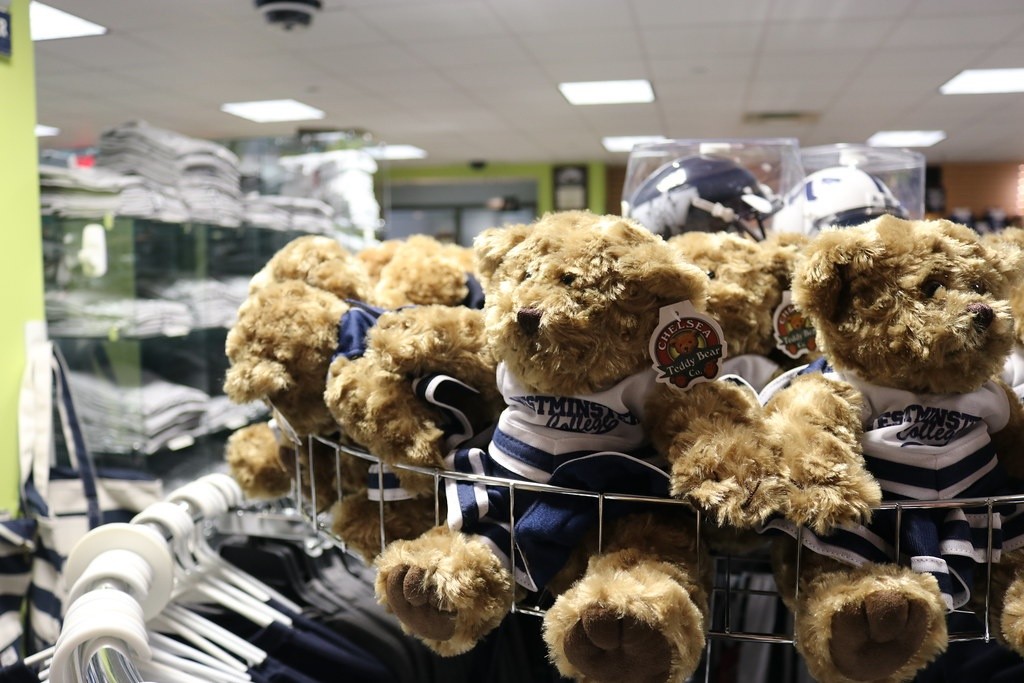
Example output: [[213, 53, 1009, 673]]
[[41, 210, 326, 469]]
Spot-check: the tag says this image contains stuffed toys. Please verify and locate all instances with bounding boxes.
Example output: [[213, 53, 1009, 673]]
[[224, 209, 1022, 683]]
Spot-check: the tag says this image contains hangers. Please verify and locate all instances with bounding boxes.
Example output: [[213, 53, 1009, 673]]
[[23, 471, 367, 683]]
